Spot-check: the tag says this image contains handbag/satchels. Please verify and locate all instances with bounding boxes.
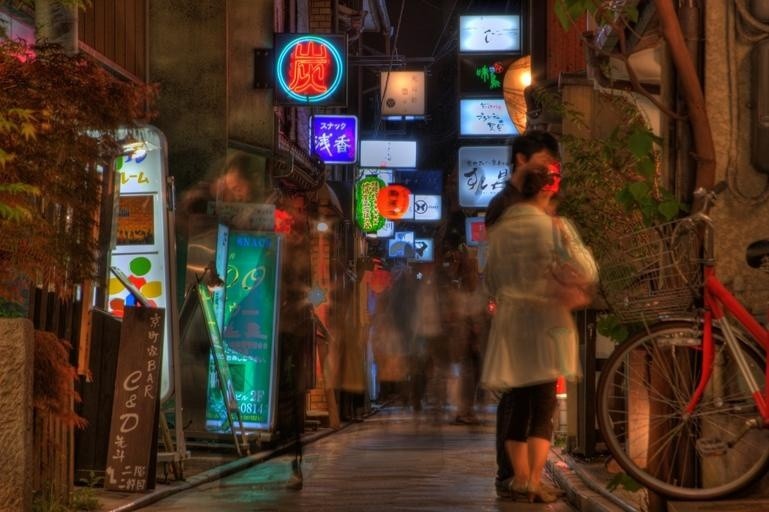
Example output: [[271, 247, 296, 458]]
[[538, 218, 591, 308]]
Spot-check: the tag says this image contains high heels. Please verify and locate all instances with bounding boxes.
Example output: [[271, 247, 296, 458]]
[[508, 480, 557, 505]]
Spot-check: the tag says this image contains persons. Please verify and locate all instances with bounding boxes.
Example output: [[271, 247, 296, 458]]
[[179, 131, 601, 504]]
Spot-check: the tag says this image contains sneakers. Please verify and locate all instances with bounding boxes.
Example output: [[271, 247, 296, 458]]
[[494, 477, 530, 503]]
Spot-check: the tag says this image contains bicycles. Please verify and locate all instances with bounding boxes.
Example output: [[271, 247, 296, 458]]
[[595, 180, 769, 502]]
